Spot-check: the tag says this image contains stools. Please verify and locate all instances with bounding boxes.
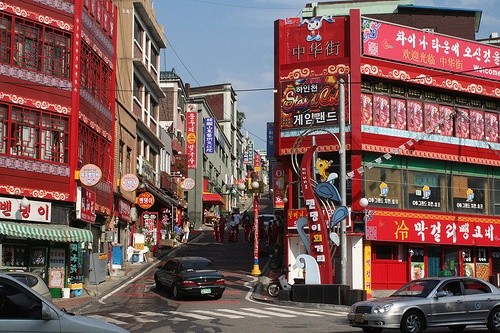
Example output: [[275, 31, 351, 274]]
[[70, 288, 82, 296]]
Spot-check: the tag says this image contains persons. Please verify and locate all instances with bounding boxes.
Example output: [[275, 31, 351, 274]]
[[173, 208, 282, 251], [442, 283, 454, 296]]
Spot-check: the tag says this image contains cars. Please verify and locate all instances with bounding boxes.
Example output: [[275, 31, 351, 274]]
[[259, 214, 282, 234], [0, 272, 52, 302], [273, 209, 284, 221], [348, 276, 500, 333], [0, 272, 131, 333], [154, 257, 226, 301]]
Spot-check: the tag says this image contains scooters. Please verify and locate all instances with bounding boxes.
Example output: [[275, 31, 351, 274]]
[[266, 264, 292, 297]]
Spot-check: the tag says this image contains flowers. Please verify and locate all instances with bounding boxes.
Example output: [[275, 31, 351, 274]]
[[160, 229, 167, 235]]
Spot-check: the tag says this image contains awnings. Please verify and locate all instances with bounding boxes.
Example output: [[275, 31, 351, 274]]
[[0, 220, 93, 242]]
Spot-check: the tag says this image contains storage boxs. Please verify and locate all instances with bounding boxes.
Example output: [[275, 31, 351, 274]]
[[68, 283, 83, 289]]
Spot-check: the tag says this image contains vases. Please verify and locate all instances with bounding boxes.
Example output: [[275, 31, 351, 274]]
[[162, 234, 165, 240]]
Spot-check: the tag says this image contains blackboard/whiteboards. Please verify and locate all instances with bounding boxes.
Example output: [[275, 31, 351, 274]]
[[48, 268, 64, 288]]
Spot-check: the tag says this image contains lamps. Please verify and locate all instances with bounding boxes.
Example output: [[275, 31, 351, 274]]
[[409, 249, 414, 258], [359, 197, 369, 207], [20, 196, 29, 208], [462, 251, 466, 258]]
